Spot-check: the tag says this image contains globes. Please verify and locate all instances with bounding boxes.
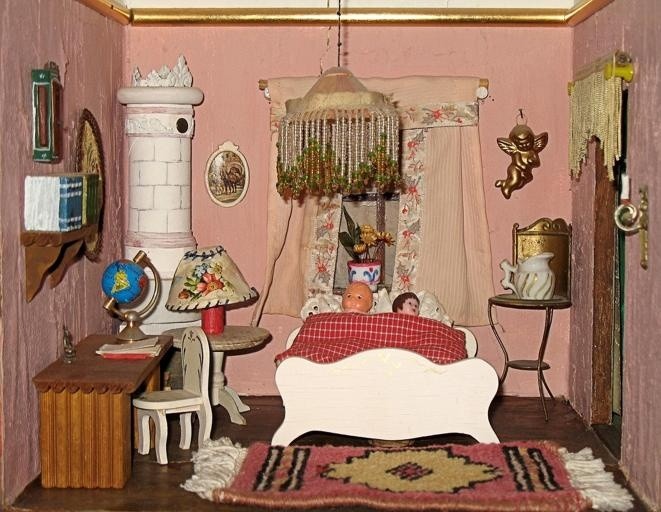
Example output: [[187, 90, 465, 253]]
[[101, 250, 163, 345]]
